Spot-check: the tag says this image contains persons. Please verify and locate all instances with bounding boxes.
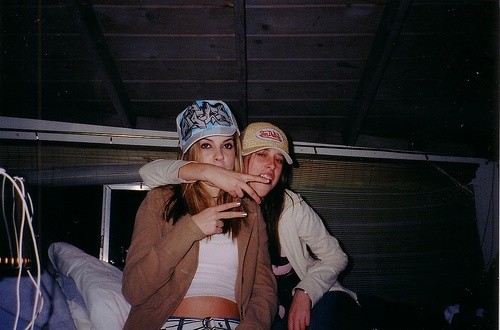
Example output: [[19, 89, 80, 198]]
[[120, 100, 279, 330], [139, 122, 367, 330]]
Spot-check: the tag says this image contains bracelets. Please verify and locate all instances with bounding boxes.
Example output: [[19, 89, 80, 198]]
[[295, 288, 309, 295]]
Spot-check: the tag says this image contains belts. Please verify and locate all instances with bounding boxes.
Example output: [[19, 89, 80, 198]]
[[160, 317, 240, 330]]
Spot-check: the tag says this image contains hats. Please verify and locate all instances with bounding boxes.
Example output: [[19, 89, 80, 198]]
[[241, 122, 293, 164], [176, 99, 240, 153]]
